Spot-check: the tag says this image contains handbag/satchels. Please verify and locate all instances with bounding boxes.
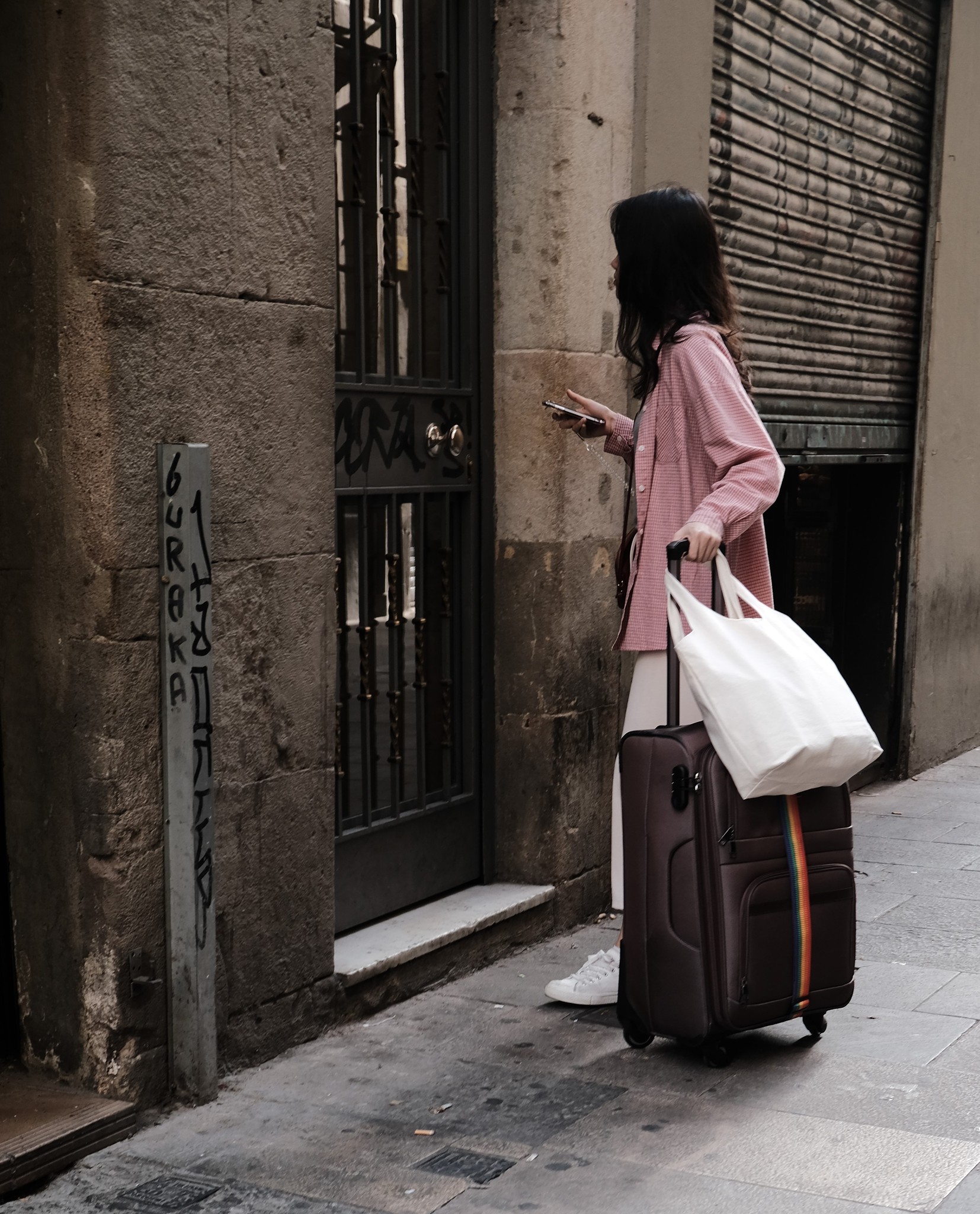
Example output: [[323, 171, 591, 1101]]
[[666, 552, 882, 796], [615, 528, 637, 608]]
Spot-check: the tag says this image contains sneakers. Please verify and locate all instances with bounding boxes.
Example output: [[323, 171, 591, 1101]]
[[545, 945, 619, 1004]]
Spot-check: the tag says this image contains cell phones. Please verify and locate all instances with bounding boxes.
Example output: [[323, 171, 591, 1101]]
[[542, 399, 607, 424]]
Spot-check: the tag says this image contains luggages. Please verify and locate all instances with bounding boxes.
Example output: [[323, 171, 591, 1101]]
[[616, 552, 855, 1047]]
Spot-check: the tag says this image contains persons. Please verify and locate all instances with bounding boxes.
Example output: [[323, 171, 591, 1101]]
[[552, 181, 786, 1006]]
